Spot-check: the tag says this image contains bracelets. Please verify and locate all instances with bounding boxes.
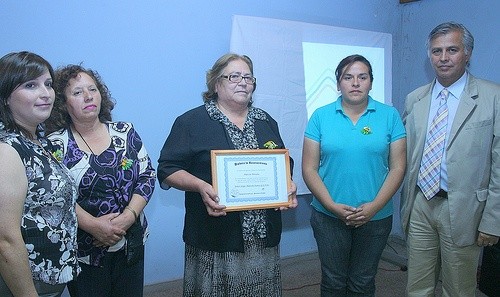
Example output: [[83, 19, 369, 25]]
[[124, 205, 139, 222]]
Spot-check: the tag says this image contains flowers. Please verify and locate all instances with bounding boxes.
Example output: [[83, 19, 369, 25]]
[[121, 156, 134, 170], [50, 150, 65, 163], [264, 140, 279, 150], [361, 125, 371, 135]]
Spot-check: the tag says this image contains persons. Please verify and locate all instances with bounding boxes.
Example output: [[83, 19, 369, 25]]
[[399, 21, 499, 297], [0, 51, 84, 297], [45, 63, 158, 297], [301, 55, 407, 297], [156, 53, 298, 297]]
